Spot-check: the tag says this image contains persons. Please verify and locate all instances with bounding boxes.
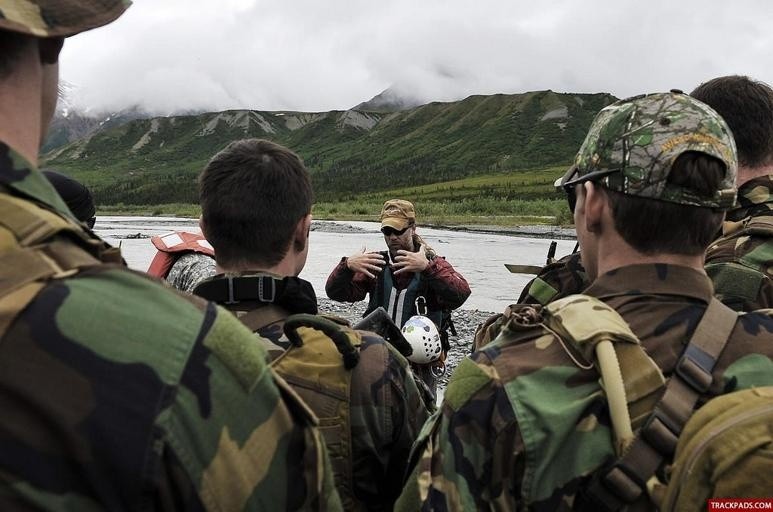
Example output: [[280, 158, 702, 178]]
[[3, 1, 773, 512]]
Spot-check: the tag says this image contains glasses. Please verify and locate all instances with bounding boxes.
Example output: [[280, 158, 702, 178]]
[[563, 168, 622, 213], [379, 227, 410, 236]]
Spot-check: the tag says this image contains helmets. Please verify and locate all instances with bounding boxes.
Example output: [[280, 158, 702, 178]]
[[399, 315, 443, 365]]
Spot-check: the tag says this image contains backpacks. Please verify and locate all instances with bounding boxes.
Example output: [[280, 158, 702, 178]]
[[535, 289, 773, 510]]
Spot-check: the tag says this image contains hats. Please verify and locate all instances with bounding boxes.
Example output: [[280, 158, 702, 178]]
[[553, 87, 740, 214], [379, 198, 417, 232]]
